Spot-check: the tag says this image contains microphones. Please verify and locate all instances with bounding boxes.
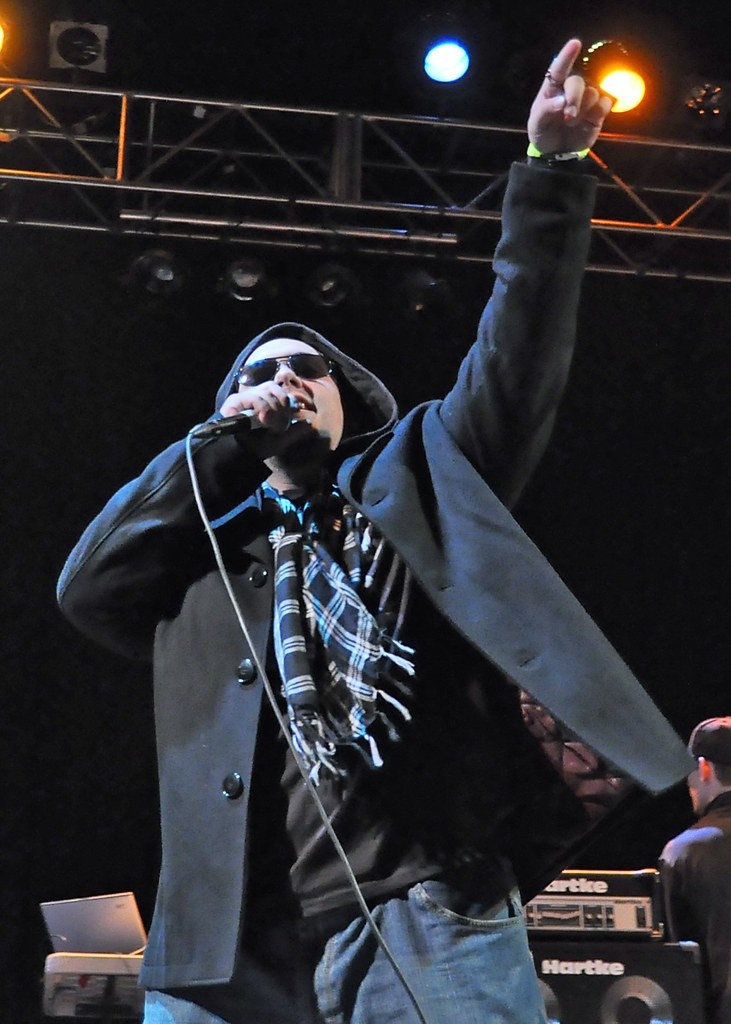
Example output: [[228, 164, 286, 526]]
[[191, 394, 299, 440]]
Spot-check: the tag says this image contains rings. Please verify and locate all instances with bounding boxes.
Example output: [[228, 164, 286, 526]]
[[543, 72, 561, 85]]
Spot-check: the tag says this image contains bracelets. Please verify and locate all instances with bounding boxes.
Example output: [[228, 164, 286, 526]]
[[526, 141, 592, 169]]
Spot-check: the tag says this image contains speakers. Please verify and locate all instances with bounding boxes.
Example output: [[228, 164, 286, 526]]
[[526, 939, 707, 1024]]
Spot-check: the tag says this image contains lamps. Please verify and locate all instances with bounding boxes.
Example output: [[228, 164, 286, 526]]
[[116, 242, 458, 325], [575, 33, 647, 113], [46, 21, 109, 72]]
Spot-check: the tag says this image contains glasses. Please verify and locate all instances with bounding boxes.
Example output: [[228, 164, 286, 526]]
[[233, 353, 335, 387]]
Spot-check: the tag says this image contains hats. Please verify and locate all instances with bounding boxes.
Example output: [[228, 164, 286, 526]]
[[688, 715, 731, 763]]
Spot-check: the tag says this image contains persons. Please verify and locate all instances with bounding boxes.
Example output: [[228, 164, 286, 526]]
[[54, 41, 696, 1024], [657, 718, 731, 1024]]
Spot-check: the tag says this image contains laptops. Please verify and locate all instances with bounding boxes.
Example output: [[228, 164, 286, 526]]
[[39, 893, 147, 956]]
